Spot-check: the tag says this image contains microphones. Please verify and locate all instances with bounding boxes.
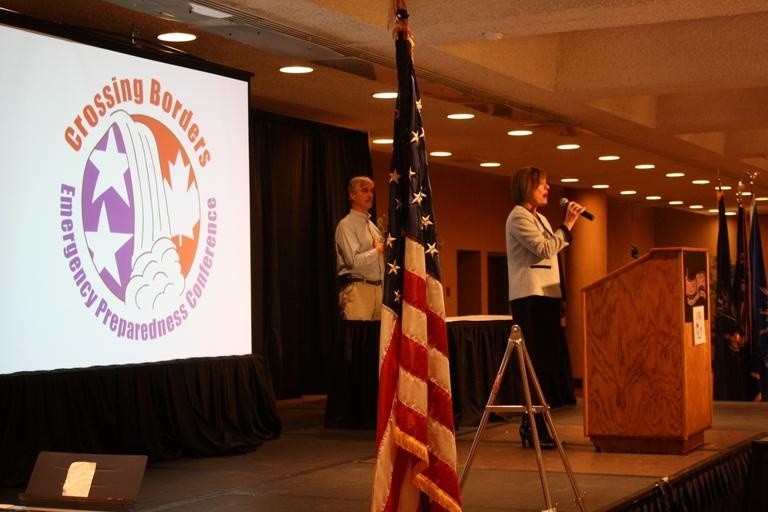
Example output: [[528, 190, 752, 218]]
[[559, 197, 595, 221]]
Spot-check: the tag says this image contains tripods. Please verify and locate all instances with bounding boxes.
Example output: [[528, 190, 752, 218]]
[[460, 324, 586, 512]]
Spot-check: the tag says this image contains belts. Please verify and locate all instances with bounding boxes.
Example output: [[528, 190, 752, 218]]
[[346, 277, 381, 286]]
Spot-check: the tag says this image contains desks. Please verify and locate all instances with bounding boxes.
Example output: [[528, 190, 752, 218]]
[[443, 313, 517, 434]]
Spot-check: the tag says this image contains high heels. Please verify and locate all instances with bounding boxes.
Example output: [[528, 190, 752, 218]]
[[519, 423, 555, 449]]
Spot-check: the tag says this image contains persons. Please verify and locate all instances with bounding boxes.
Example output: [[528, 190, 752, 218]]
[[504, 166, 588, 450], [334, 175, 385, 321]]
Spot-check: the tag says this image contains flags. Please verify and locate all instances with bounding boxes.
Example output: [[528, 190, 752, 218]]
[[712, 192, 767, 402], [369, 37, 462, 512]]
